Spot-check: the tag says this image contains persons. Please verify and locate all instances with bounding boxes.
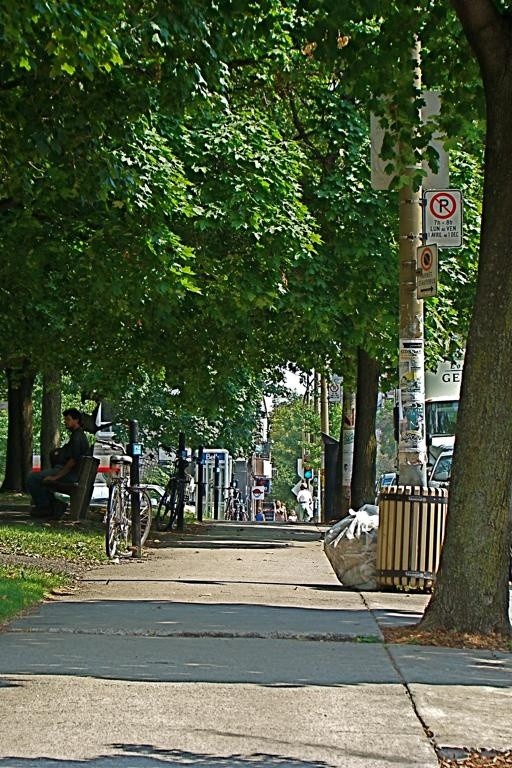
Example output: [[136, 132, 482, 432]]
[[288, 509, 298, 522], [255, 506, 265, 521], [297, 482, 314, 522], [24, 407, 90, 519], [274, 499, 288, 521]]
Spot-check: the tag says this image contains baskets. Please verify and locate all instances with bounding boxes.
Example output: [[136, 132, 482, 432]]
[[124, 458, 157, 485]]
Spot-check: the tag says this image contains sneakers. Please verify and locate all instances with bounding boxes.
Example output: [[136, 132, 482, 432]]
[[29, 501, 67, 519]]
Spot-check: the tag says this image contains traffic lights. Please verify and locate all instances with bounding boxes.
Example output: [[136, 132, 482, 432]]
[[304, 454, 312, 480]]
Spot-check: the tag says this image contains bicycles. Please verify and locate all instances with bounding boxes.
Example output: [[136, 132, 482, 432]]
[[94, 436, 160, 562], [154, 443, 202, 533]]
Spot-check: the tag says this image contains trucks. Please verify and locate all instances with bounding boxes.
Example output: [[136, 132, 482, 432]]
[[389, 347, 468, 488]]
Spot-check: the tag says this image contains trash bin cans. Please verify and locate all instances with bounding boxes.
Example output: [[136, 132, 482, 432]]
[[376, 485, 449, 593]]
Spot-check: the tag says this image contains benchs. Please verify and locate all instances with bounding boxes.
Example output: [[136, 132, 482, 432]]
[[56, 456, 100, 521]]
